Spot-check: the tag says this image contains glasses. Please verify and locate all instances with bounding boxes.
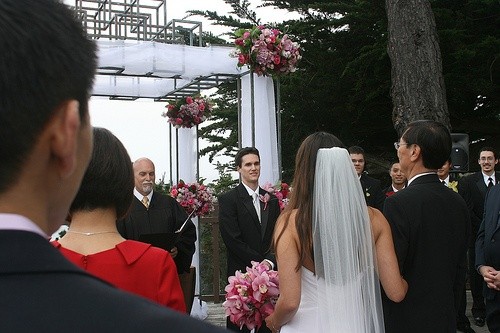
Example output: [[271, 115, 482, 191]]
[[394, 142, 408, 150]]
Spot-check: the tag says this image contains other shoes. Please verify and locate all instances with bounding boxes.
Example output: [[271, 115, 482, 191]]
[[472, 311, 485, 328]]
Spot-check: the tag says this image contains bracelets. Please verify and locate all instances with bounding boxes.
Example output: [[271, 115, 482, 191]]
[[271, 316, 281, 331]]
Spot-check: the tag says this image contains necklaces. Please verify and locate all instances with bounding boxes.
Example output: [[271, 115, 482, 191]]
[[66, 231, 119, 236]]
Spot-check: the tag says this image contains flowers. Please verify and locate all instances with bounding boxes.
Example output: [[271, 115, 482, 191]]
[[170, 180, 217, 219], [162, 93, 214, 130], [232, 24, 302, 79], [264, 180, 294, 212], [222, 260, 278, 333], [259, 192, 270, 208], [445, 180, 459, 193]]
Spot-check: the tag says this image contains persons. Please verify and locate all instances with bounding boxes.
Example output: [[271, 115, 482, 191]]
[[0, 0, 500, 333]]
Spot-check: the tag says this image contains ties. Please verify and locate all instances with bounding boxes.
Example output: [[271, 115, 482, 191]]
[[142, 196, 149, 209], [442, 181, 445, 185], [252, 193, 261, 223], [487, 178, 494, 192]]
